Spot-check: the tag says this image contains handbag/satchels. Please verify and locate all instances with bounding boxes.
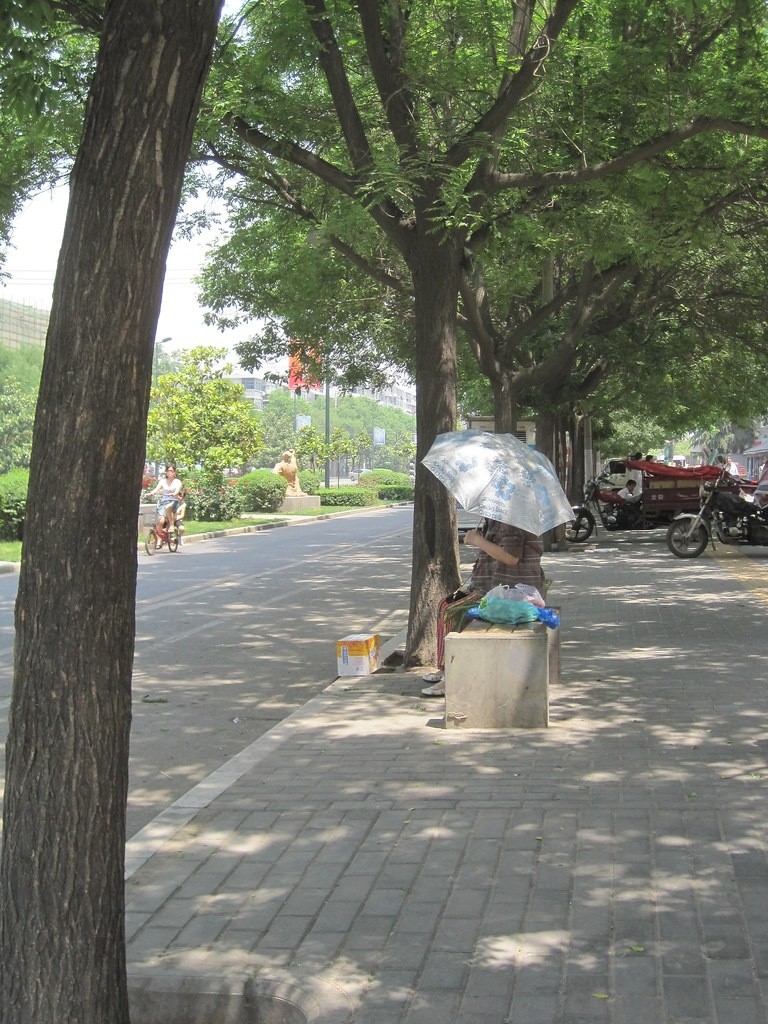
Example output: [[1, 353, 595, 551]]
[[446, 590, 468, 603], [469, 582, 558, 629]]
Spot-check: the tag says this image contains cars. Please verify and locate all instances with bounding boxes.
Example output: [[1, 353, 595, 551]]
[[349, 469, 370, 481]]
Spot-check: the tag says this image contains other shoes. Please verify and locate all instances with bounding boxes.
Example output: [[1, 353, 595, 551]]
[[167, 524, 175, 533], [421, 675, 445, 696], [156, 538, 162, 548], [423, 672, 444, 682], [177, 536, 183, 546]]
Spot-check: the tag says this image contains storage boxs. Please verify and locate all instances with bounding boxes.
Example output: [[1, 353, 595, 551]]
[[337, 631, 381, 676]]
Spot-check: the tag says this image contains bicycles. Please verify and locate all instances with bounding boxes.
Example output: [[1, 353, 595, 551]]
[[139, 496, 181, 556]]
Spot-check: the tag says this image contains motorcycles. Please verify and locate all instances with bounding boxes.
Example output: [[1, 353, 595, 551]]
[[665, 454, 768, 559], [564, 459, 758, 542]]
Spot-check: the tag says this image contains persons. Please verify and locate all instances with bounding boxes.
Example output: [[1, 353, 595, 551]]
[[272, 448, 297, 484], [616, 452, 655, 504], [723, 457, 739, 475], [147, 465, 188, 549], [421, 518, 543, 696]]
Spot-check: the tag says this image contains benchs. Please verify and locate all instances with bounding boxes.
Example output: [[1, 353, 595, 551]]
[[444, 604, 565, 732]]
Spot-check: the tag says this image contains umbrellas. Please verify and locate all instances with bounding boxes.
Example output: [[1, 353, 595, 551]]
[[420, 428, 577, 538]]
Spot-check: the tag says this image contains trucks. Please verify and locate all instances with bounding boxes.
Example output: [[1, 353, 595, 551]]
[[599, 459, 631, 492]]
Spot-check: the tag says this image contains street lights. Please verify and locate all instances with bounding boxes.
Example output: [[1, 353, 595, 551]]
[[156, 338, 173, 388]]
